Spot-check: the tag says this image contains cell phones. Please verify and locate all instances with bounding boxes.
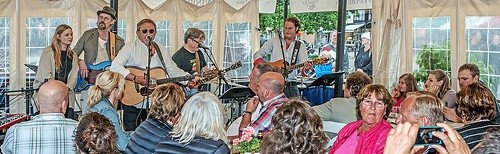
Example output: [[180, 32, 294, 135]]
[[413, 126, 443, 146]]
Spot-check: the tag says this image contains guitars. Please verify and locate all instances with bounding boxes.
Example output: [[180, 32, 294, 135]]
[[248, 55, 331, 94], [189, 61, 242, 88], [73, 61, 112, 92], [113, 66, 218, 107]]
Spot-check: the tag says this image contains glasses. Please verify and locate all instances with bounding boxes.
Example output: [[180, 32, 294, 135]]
[[99, 16, 112, 21], [363, 99, 384, 108], [138, 29, 155, 33]]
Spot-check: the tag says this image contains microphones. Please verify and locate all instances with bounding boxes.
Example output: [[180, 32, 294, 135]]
[[198, 44, 211, 50], [278, 30, 282, 39], [145, 35, 155, 45]]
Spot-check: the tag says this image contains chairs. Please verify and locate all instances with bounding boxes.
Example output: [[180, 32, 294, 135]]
[[322, 120, 348, 143]]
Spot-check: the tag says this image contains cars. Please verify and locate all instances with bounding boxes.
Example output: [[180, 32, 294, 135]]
[[343, 40, 355, 51]]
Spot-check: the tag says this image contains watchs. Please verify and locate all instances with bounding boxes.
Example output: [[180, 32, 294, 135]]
[[133, 75, 137, 83]]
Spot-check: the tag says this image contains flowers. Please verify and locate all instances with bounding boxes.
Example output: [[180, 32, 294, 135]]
[[233, 126, 262, 154]]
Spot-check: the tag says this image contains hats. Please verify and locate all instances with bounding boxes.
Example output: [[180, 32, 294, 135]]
[[96, 6, 117, 20]]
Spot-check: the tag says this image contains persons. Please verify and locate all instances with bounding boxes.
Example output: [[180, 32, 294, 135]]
[[71, 71, 129, 154], [72, 6, 125, 85], [318, 30, 349, 76], [443, 64, 499, 123], [124, 83, 231, 154], [312, 68, 374, 123], [253, 16, 315, 98], [451, 82, 500, 154], [382, 91, 472, 154], [0, 80, 83, 154], [226, 62, 328, 154], [323, 84, 394, 154], [355, 32, 372, 78], [389, 73, 419, 112], [425, 69, 457, 108], [32, 24, 79, 119], [171, 28, 214, 94], [109, 19, 170, 131]]
